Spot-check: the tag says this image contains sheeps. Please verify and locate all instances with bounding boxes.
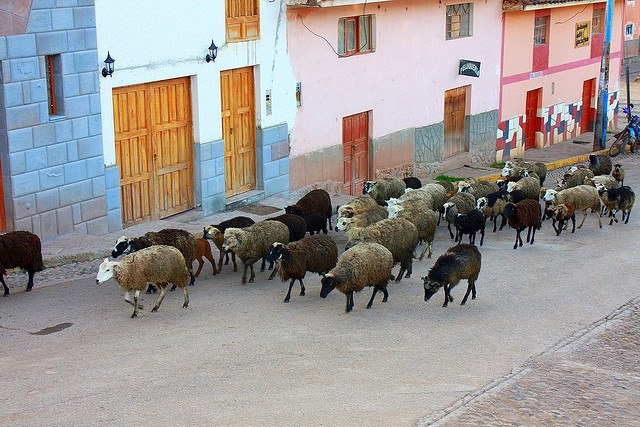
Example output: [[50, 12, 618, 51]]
[[301, 213, 327, 236], [0, 231, 45, 295], [403, 177, 422, 190], [540, 186, 566, 221], [362, 178, 406, 205], [202, 217, 256, 272], [611, 163, 625, 188], [594, 181, 635, 225], [456, 179, 499, 197], [477, 191, 511, 232], [521, 162, 547, 187], [320, 241, 394, 312], [543, 185, 602, 229], [412, 183, 447, 226], [545, 202, 576, 235], [434, 181, 455, 197], [386, 203, 438, 259], [222, 220, 291, 283], [504, 199, 542, 249], [260, 214, 307, 271], [500, 166, 519, 179], [421, 242, 481, 307], [587, 152, 612, 176], [336, 196, 377, 222], [507, 176, 540, 203], [112, 229, 196, 294], [583, 175, 619, 218], [517, 166, 529, 178], [439, 193, 475, 239], [267, 234, 338, 302], [384, 189, 434, 212], [503, 158, 525, 171], [193, 239, 217, 277], [334, 205, 389, 234], [556, 170, 590, 191], [566, 165, 588, 172], [285, 190, 333, 232], [344, 217, 420, 284], [522, 169, 540, 184], [94, 245, 191, 317]]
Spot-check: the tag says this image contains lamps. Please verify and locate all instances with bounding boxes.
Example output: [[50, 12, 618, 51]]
[[206, 39, 218, 64], [102, 52, 115, 77]]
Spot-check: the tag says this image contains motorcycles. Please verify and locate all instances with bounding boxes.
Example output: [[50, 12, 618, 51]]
[[609, 104, 640, 158]]
